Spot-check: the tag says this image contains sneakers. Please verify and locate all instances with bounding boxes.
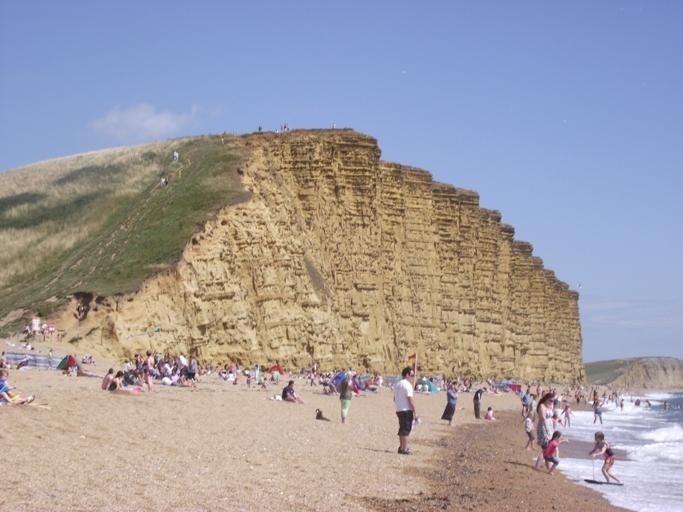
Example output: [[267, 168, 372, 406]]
[[398, 446, 412, 454]]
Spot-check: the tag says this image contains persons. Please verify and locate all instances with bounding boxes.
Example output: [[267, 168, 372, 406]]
[[165, 176, 168, 185], [393, 365, 672, 487], [20, 322, 96, 376], [101, 349, 384, 424], [1, 352, 35, 405], [161, 176, 165, 186]]
